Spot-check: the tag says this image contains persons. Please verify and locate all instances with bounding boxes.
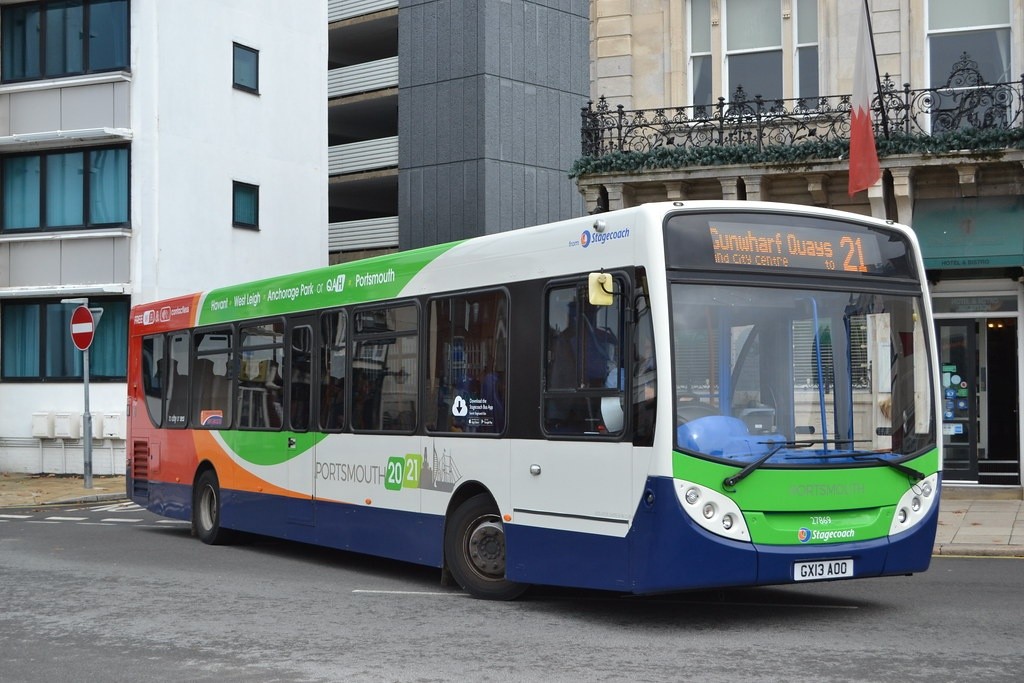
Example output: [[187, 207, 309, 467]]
[[352, 368, 463, 432], [605, 334, 693, 405]]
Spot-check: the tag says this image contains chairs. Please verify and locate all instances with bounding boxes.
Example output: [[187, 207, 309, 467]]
[[154, 358, 502, 434]]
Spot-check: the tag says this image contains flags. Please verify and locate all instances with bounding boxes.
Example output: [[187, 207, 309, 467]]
[[848, 0, 880, 197]]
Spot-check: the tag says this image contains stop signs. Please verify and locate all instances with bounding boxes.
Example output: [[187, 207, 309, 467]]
[[70, 305, 94, 351]]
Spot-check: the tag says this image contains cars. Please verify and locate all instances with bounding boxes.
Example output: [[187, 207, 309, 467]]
[[126, 200, 946, 603]]
